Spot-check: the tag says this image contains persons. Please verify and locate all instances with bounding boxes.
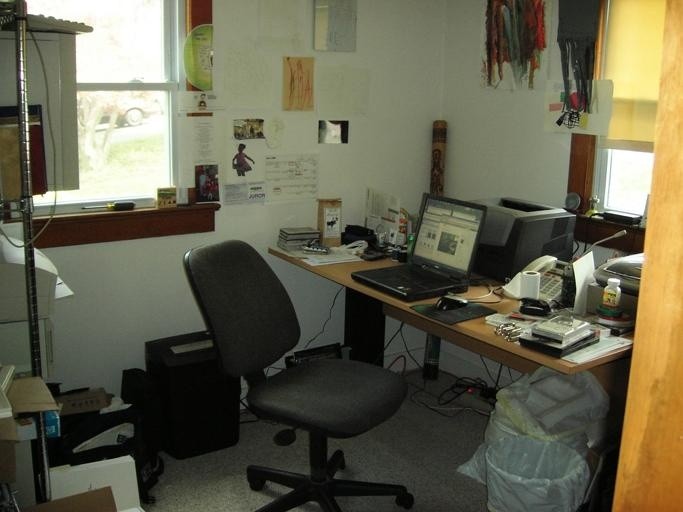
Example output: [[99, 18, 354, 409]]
[[199, 171, 218, 200], [232, 143, 255, 176]]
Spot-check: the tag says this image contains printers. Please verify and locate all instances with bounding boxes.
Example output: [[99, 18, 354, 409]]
[[463, 194, 577, 290]]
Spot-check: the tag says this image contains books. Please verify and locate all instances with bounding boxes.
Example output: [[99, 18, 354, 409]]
[[277, 226, 321, 250]]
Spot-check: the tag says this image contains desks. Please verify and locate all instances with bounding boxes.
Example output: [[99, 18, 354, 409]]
[[269, 247, 635, 377]]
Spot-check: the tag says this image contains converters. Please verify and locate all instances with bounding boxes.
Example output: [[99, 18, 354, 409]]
[[481, 386, 494, 397]]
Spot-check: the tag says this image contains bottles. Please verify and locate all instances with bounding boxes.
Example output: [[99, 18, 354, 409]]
[[406, 233, 416, 262], [599, 277, 622, 323]]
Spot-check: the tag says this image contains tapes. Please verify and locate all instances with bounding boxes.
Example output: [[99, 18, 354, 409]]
[[596, 303, 624, 317]]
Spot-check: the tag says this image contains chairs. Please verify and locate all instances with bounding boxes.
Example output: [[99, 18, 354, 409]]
[[185, 239, 414, 512]]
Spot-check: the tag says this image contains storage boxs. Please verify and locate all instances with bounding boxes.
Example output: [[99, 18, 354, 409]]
[[0, 375, 59, 483]]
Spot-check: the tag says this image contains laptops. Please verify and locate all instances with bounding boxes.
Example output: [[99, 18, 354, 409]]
[[350, 191, 489, 303]]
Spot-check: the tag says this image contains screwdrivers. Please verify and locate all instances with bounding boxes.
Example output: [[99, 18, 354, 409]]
[[80, 201, 136, 211]]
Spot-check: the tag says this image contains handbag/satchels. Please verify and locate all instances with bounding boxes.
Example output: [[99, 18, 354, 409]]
[[59, 410, 164, 502]]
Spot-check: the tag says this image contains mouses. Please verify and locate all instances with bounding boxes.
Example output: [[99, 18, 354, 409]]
[[436, 293, 468, 312]]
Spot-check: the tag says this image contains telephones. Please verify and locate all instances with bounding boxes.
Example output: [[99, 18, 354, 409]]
[[502, 255, 573, 305]]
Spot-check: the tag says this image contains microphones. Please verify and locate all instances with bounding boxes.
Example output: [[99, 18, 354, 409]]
[[580, 227, 629, 261]]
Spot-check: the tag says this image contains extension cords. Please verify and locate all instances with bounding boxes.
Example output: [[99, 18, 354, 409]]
[[460, 389, 493, 416]]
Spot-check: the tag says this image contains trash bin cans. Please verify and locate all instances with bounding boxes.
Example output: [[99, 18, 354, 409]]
[[485, 437, 590, 512]]
